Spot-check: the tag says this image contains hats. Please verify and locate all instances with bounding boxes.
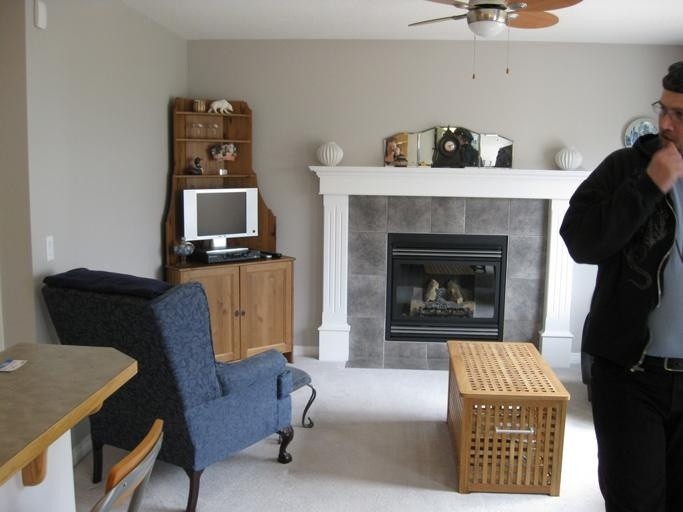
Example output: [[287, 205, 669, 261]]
[[662, 61, 683, 93]]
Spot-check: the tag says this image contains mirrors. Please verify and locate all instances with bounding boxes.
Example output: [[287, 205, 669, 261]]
[[381, 126, 514, 170]]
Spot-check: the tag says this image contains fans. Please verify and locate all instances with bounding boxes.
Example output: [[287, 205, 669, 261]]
[[408, 0, 584, 33]]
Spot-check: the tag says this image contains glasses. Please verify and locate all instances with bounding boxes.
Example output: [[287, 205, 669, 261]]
[[652, 100, 683, 122]]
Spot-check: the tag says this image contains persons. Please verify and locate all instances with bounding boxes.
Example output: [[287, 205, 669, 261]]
[[559, 60, 681, 512], [385, 127, 511, 167]]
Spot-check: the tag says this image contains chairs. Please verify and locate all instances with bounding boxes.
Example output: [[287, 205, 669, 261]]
[[90, 418, 168, 511], [41, 268, 316, 512]]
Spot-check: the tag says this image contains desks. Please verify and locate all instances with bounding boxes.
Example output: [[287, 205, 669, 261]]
[[445, 341, 572, 497], [0, 342, 136, 497]]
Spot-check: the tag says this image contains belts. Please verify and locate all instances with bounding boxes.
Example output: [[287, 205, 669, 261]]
[[639, 354, 682, 372]]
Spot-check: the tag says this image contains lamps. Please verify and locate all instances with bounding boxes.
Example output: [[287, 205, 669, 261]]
[[465, 0, 509, 43]]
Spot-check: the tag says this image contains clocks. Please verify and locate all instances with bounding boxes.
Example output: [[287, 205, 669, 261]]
[[432, 124, 464, 170]]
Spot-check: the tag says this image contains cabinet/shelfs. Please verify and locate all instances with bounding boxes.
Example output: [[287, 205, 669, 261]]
[[163, 251, 295, 365], [161, 94, 278, 259]]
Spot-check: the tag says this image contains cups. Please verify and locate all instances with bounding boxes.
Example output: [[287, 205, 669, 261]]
[[190, 122, 220, 141]]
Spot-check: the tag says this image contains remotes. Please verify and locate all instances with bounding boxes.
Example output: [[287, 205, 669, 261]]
[[261, 253, 273, 259], [261, 252, 281, 257]]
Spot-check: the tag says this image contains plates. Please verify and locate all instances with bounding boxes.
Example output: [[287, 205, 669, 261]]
[[624, 116, 660, 150]]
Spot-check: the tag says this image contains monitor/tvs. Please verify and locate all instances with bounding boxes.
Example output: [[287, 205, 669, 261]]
[[176, 188, 258, 254]]
